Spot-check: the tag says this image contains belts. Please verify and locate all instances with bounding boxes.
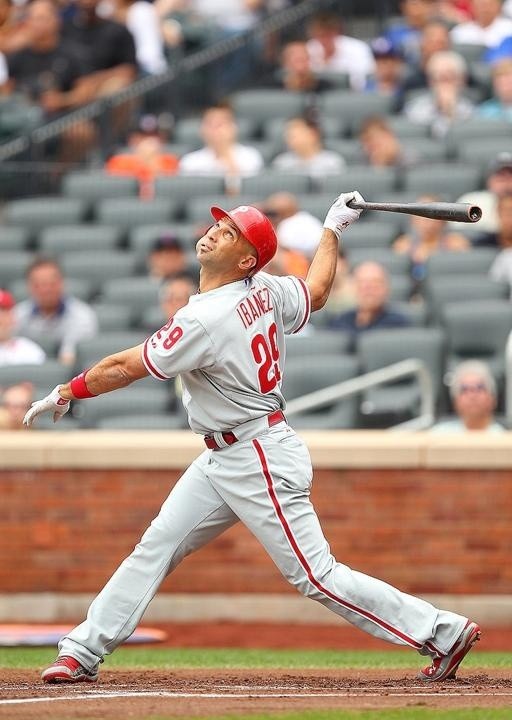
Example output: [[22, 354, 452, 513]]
[[204, 410, 284, 449]]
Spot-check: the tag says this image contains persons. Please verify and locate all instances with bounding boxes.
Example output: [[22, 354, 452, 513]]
[[157, 271, 200, 322], [4, 386, 36, 428], [2, 4, 512, 301], [21, 190, 481, 686], [11, 256, 98, 364], [427, 359, 505, 434], [324, 258, 412, 352], [0, 293, 48, 365]]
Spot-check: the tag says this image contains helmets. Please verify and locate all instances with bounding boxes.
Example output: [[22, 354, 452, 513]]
[[210, 205, 278, 279]]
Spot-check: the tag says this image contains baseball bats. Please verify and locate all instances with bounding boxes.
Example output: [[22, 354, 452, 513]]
[[333, 199, 482, 222]]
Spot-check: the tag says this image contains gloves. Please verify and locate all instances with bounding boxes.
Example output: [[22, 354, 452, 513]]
[[22, 384, 71, 430], [323, 190, 366, 241]]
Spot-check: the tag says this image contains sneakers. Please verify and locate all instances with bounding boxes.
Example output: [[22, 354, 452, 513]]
[[416, 619, 482, 682], [41, 656, 98, 682]]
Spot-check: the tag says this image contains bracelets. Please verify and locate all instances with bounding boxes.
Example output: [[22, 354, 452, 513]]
[[70, 368, 100, 399]]
